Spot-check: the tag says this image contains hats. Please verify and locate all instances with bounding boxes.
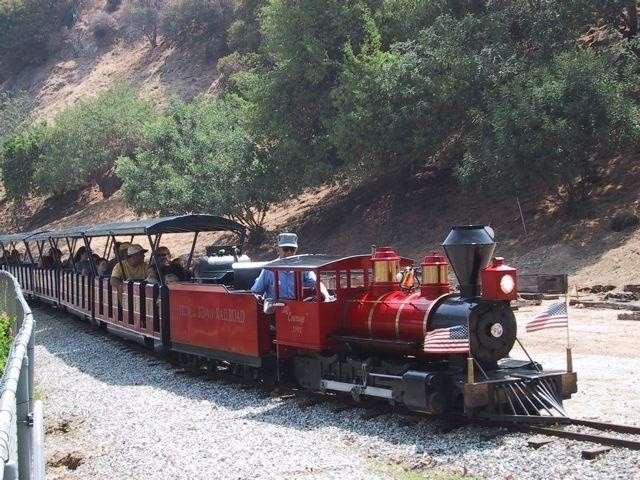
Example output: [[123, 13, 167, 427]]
[[278, 233, 299, 248], [113, 242, 147, 256]]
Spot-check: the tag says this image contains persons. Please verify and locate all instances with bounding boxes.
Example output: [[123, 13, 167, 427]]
[[0, 241, 193, 307], [248, 232, 336, 303]]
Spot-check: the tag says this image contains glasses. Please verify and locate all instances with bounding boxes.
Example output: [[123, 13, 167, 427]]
[[282, 246, 297, 252]]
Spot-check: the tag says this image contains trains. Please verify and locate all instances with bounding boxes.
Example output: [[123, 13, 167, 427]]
[[1, 205, 579, 433]]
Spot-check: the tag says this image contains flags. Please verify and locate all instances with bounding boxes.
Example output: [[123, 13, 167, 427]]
[[524, 300, 568, 333], [423, 323, 471, 357]]
